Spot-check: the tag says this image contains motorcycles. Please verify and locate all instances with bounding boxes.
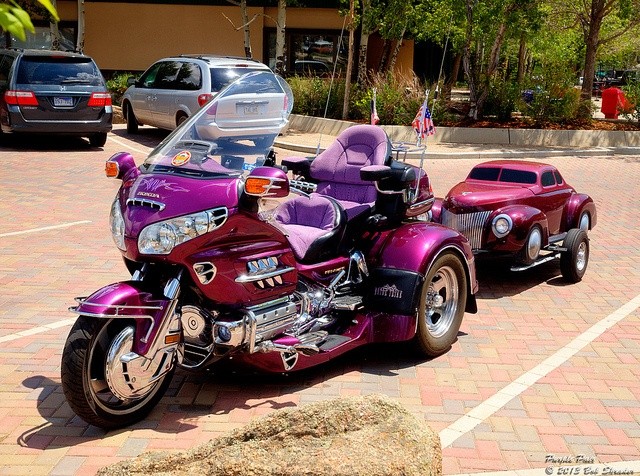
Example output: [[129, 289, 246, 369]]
[[60, 69, 481, 429]]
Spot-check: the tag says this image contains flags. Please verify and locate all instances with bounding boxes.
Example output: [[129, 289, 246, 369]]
[[411, 98, 436, 140], [370, 96, 380, 125]]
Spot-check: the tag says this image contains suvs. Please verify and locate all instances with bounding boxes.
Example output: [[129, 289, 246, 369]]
[[120, 53, 290, 148], [0, 46, 113, 147]]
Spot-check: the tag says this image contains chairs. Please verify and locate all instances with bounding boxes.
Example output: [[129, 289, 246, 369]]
[[267, 195, 348, 264], [310, 124, 393, 253]]
